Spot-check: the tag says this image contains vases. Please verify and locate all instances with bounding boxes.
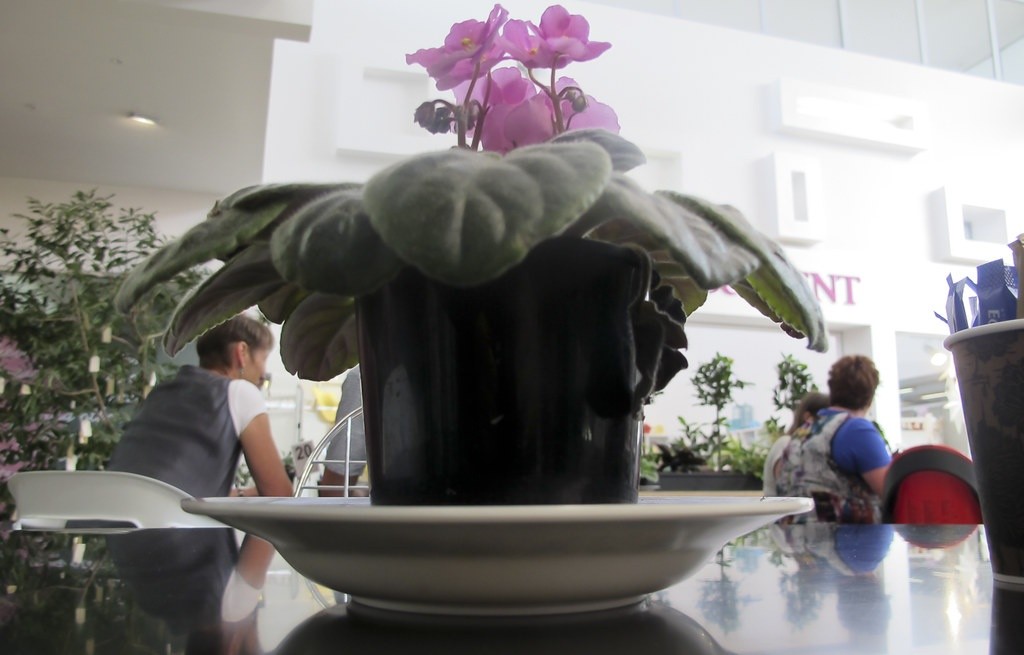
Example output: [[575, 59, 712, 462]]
[[352, 232, 647, 507]]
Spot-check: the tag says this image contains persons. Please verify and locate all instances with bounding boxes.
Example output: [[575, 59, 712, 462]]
[[815, 355, 892, 524], [795, 523, 894, 637], [763, 393, 828, 491], [104, 529, 274, 655], [317, 369, 369, 497], [110, 315, 292, 498]]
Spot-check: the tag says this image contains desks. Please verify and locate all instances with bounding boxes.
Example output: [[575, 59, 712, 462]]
[[0, 522, 1024, 655]]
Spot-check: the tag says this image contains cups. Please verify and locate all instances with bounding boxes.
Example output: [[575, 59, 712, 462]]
[[943, 319, 1024, 585]]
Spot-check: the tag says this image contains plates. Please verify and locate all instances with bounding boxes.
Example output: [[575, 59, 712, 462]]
[[180, 492, 816, 616]]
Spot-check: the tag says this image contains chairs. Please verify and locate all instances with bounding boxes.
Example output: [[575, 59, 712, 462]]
[[2, 463, 234, 537]]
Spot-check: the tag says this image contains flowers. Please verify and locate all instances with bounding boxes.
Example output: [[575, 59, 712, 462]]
[[110, 0, 833, 420]]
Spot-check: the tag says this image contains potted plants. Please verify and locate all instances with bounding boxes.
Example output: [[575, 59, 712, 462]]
[[652, 354, 758, 492]]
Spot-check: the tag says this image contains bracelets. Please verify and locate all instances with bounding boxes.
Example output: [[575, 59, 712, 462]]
[[238, 490, 243, 497]]
